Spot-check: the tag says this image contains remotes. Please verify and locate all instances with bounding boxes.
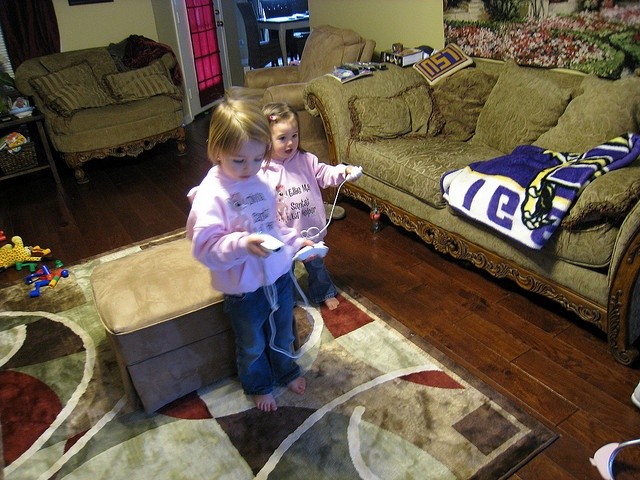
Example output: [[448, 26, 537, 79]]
[[359, 61, 375, 71], [371, 62, 387, 69], [350, 63, 363, 71]]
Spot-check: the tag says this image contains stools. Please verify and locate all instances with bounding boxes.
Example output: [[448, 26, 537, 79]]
[[89, 237, 237, 415]]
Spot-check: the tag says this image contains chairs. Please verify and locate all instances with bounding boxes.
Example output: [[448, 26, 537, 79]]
[[257, 0, 297, 67], [235, 0, 297, 70]]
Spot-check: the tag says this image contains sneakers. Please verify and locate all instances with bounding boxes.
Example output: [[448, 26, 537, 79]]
[[324, 200, 345, 218]]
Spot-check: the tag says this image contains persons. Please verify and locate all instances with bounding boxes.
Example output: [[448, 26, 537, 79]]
[[256, 101, 357, 311], [186, 98, 313, 411]]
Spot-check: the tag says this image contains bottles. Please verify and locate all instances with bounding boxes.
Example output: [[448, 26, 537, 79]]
[[368, 203, 381, 234]]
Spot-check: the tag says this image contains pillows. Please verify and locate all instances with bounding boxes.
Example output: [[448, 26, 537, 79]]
[[27, 59, 117, 118], [413, 43, 473, 86], [431, 67, 499, 142], [467, 58, 574, 156], [560, 167, 640, 229], [39, 47, 119, 82], [102, 59, 183, 103], [530, 73, 640, 154], [345, 82, 446, 160]]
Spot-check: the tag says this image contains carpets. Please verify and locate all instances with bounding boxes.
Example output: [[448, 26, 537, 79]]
[[0, 226, 559, 480]]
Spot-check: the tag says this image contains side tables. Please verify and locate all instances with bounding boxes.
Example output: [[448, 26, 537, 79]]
[[0, 113, 61, 184]]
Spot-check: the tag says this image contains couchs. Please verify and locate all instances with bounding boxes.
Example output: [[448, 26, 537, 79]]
[[244, 25, 376, 165], [14, 34, 189, 185], [302, 58, 640, 365]]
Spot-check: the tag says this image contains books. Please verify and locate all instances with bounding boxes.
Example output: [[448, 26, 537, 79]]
[[382, 47, 424, 67]]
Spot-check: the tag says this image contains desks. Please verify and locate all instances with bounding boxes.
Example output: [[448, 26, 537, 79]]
[[257, 14, 310, 66]]
